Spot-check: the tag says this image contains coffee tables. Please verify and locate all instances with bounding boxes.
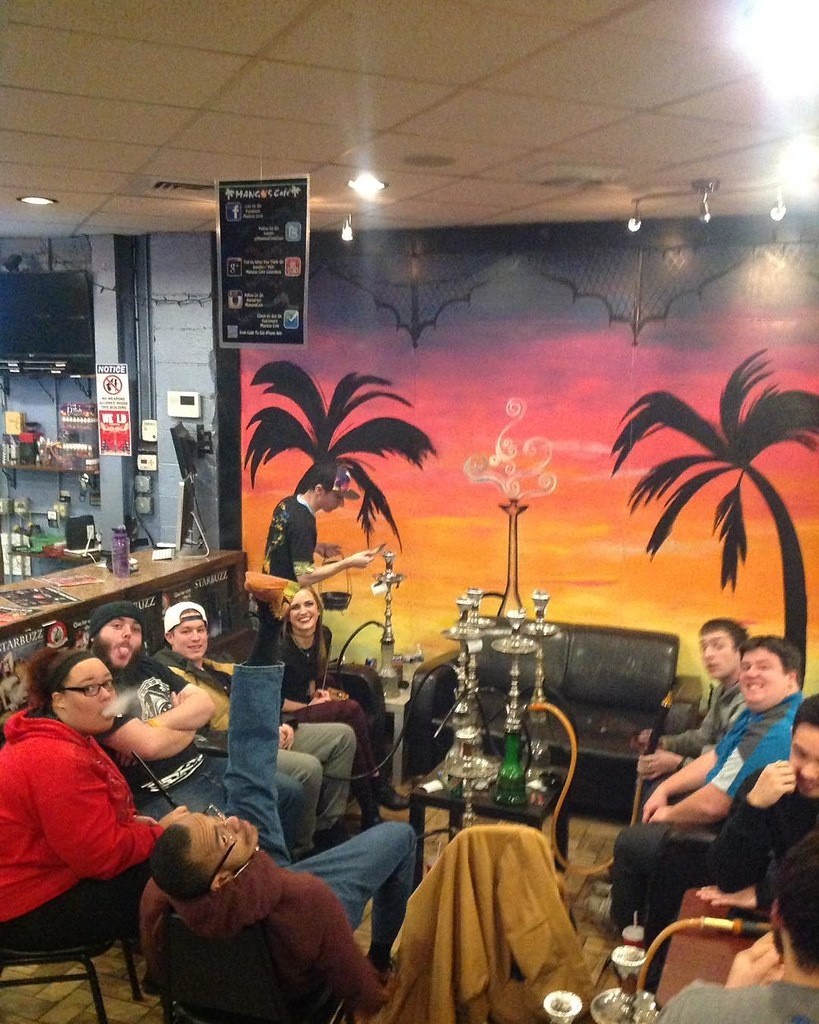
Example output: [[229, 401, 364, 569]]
[[410, 756, 569, 891]]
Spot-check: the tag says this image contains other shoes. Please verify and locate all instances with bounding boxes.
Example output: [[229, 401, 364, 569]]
[[360, 813, 383, 832], [584, 896, 620, 927], [587, 874, 614, 898], [368, 954, 397, 984], [312, 831, 354, 851], [374, 787, 410, 811]]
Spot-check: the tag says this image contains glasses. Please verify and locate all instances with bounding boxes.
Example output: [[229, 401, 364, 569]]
[[64, 679, 119, 697], [204, 803, 238, 878]]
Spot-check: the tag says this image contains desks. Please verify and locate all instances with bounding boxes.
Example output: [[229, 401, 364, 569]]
[[656, 887, 773, 1011]]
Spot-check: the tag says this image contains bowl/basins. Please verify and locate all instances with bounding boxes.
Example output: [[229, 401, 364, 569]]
[[321, 592, 351, 610]]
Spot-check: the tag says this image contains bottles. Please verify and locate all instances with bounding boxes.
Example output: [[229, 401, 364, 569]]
[[110, 528, 130, 578], [413, 643, 424, 673], [0, 497, 29, 514], [53, 491, 70, 518], [19, 421, 41, 466]]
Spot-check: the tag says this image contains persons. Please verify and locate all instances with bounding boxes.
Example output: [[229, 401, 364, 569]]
[[585, 633, 804, 932], [643, 693, 819, 993], [636, 621, 750, 811], [141, 571, 417, 1018], [282, 586, 411, 829], [265, 463, 378, 587], [651, 832, 819, 1024], [0, 649, 188, 951], [156, 600, 356, 859], [91, 602, 302, 854]]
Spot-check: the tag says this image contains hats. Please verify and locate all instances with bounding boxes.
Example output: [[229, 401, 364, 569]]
[[164, 601, 208, 636], [310, 464, 360, 499], [89, 601, 145, 638]]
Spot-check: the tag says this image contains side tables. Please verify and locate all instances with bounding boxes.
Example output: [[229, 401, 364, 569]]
[[383, 671, 414, 786]]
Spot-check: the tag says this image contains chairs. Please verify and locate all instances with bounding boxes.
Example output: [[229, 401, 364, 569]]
[[351, 824, 598, 1024], [162, 911, 354, 1024], [0, 931, 142, 1024]]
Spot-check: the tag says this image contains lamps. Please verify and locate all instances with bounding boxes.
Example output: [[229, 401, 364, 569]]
[[698, 190, 711, 224], [628, 200, 642, 232], [341, 215, 353, 241], [769, 186, 787, 222]]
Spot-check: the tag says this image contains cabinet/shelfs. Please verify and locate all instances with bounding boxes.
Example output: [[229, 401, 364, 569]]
[[0, 463, 101, 561]]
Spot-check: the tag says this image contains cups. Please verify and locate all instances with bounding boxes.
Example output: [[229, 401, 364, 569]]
[[622, 925, 644, 946]]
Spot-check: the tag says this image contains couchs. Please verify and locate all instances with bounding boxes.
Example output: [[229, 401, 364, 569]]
[[407, 615, 703, 820], [204, 618, 388, 807], [514, 673, 521, 678]]
